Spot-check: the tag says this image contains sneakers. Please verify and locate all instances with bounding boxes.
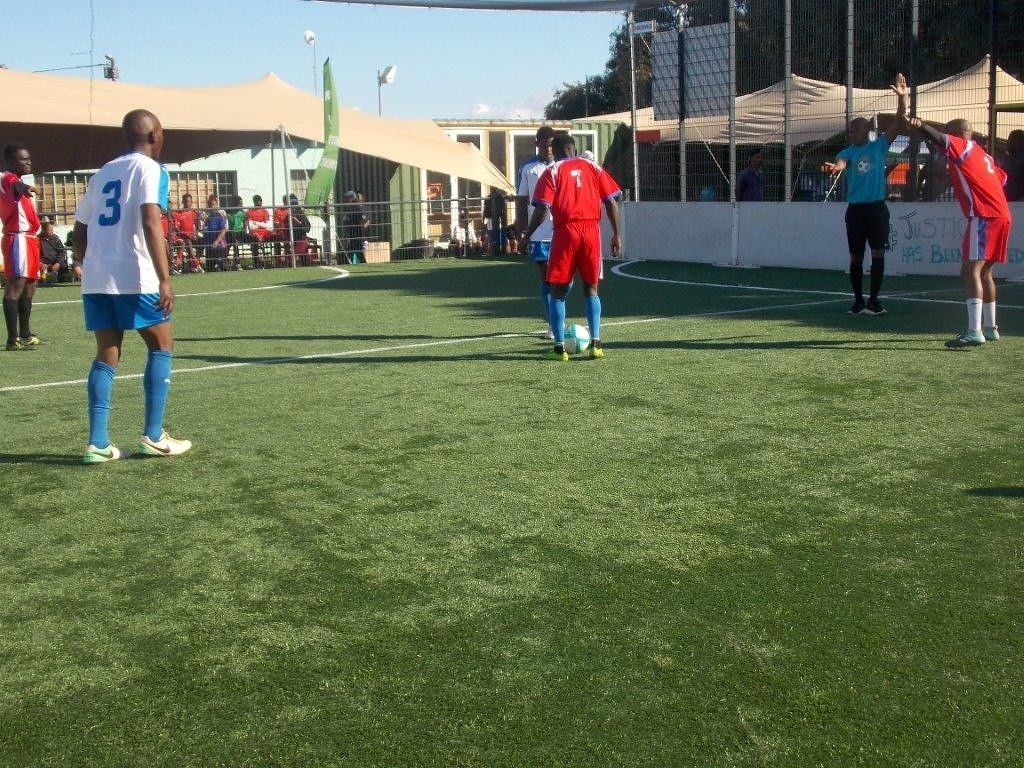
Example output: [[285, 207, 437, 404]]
[[846, 301, 866, 314], [982, 325, 999, 340], [589, 346, 604, 357], [139, 428, 192, 455], [944, 329, 986, 349], [867, 299, 888, 314], [170, 251, 313, 276], [544, 350, 569, 362], [19, 334, 51, 345], [83, 442, 134, 463], [7, 336, 37, 350], [544, 331, 555, 340]]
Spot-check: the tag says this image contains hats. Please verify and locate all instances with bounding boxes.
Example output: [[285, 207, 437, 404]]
[[344, 191, 357, 200]]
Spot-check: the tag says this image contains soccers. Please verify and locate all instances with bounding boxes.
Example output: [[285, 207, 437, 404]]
[[563, 324, 591, 354], [856, 157, 873, 177]]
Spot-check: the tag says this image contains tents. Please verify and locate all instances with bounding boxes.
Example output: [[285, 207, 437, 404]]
[[571, 52, 1024, 200], [0, 68, 517, 269]]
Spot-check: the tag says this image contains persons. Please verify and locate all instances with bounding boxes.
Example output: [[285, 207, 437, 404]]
[[824, 73, 908, 316], [0, 141, 51, 352], [518, 134, 621, 362], [902, 115, 1012, 347], [511, 126, 574, 340], [70, 109, 191, 464], [36, 186, 532, 286], [916, 148, 948, 203], [1001, 130, 1024, 203], [734, 149, 765, 202]]
